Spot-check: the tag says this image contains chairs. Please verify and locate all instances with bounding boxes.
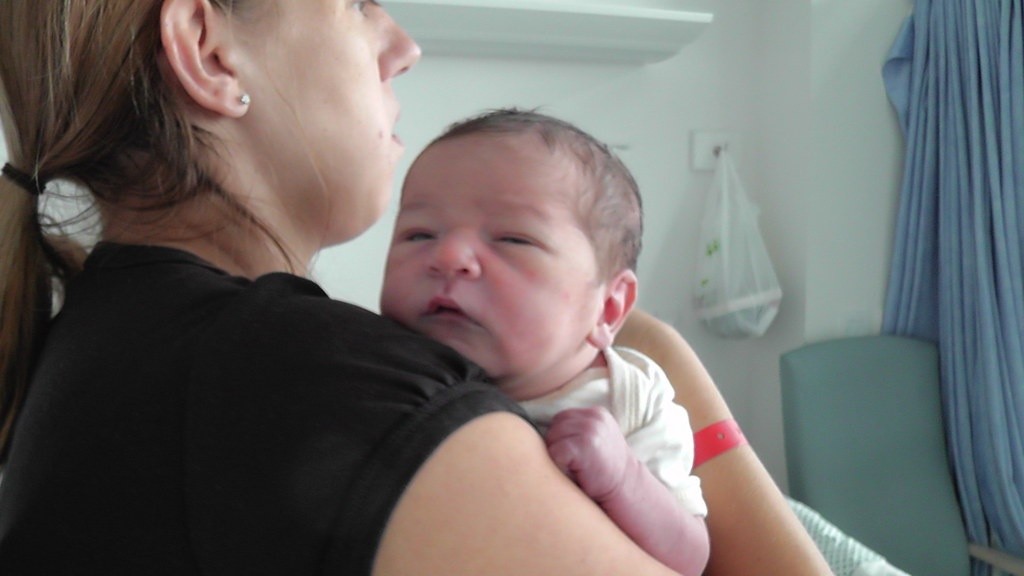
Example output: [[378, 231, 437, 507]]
[[768, 333, 1024, 576]]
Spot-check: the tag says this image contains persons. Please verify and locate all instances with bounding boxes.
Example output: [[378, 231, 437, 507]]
[[381, 109, 710, 576], [0, 0, 836, 576]]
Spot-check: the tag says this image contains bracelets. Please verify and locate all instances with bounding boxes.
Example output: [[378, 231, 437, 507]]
[[693, 416, 745, 468]]
